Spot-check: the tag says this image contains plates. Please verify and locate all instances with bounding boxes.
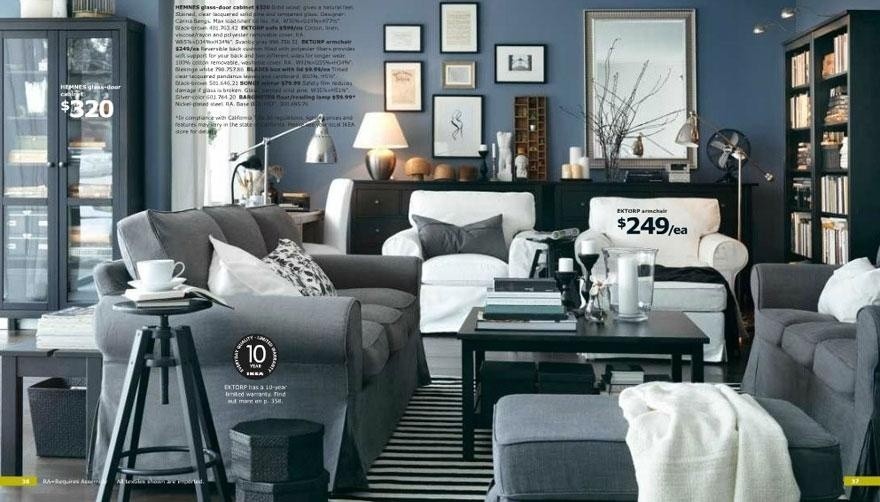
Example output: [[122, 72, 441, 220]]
[[125, 276, 188, 291]]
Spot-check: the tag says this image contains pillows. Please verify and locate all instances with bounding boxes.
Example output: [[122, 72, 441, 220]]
[[206, 232, 302, 296], [411, 212, 510, 264], [261, 237, 339, 296], [816, 256, 880, 322]]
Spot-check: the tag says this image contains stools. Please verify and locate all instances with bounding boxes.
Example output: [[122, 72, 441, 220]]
[[0, 337, 103, 475]]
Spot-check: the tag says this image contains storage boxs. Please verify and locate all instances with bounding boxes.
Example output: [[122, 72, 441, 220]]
[[234, 468, 329, 501], [5, 212, 48, 257], [29, 377, 85, 461], [5, 268, 48, 303], [230, 419, 324, 481]]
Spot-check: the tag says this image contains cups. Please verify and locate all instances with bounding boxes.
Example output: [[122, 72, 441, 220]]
[[237, 192, 271, 211], [560, 146, 591, 180], [136, 258, 187, 281], [530, 124, 536, 132], [601, 246, 659, 322]]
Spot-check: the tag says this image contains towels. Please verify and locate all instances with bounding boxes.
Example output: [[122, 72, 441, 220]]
[[619, 375, 804, 501]]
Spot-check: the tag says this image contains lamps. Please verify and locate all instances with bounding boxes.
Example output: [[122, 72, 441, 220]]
[[751, 7, 839, 38], [633, 130, 674, 157], [229, 153, 265, 206], [227, 114, 338, 209], [352, 111, 411, 182], [674, 109, 776, 341]]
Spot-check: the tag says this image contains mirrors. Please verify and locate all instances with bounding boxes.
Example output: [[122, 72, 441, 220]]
[[581, 8, 701, 172]]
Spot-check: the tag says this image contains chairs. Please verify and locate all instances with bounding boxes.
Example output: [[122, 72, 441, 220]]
[[303, 177, 355, 255]]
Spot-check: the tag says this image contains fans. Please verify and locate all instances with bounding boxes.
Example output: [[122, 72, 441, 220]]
[[706, 128, 752, 184]]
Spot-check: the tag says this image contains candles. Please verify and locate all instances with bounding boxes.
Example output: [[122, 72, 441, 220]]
[[491, 143, 496, 157], [478, 143, 487, 151], [561, 146, 591, 179]]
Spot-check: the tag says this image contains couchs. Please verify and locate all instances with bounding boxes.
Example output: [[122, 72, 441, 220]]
[[89, 198, 432, 492], [574, 196, 752, 367], [380, 189, 553, 338], [485, 388, 846, 500], [742, 243, 880, 501]]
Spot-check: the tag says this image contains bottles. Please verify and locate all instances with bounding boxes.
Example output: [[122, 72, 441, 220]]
[[260, 181, 281, 205]]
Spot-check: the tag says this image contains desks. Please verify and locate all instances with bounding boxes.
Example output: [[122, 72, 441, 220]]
[[283, 207, 325, 246], [98, 294, 232, 501]]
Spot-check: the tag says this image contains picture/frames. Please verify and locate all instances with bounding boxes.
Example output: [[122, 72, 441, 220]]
[[440, 2, 480, 54], [383, 24, 424, 53], [383, 60, 423, 113], [431, 94, 486, 159], [441, 60, 476, 90], [494, 43, 547, 84]]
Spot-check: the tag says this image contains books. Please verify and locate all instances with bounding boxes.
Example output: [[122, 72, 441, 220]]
[[132, 297, 208, 308], [34, 302, 101, 351], [599, 372, 638, 392], [785, 34, 853, 266], [4, 122, 115, 200], [474, 277, 576, 330], [122, 282, 236, 311], [606, 362, 647, 385]]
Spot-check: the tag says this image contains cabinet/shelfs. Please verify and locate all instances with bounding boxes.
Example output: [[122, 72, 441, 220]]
[[349, 180, 550, 256], [780, 8, 879, 268], [1, 18, 147, 332], [511, 96, 548, 180], [552, 180, 760, 269]]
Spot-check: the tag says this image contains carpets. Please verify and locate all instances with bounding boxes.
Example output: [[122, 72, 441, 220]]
[[329, 371, 497, 500]]
